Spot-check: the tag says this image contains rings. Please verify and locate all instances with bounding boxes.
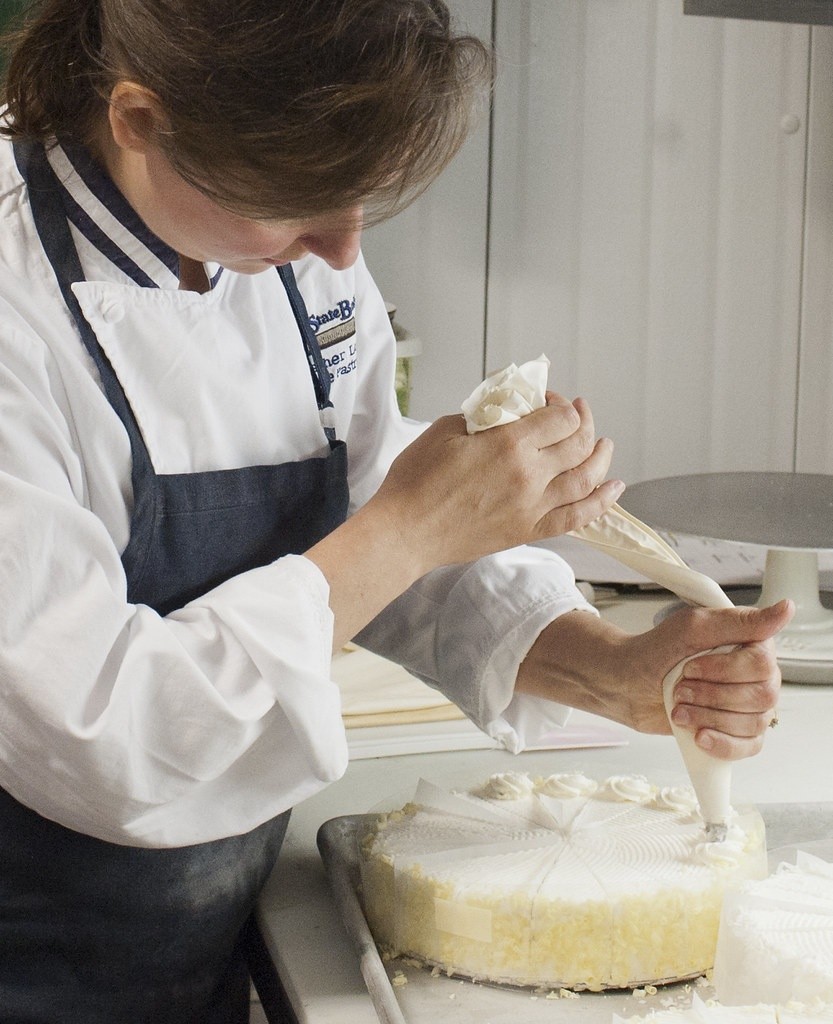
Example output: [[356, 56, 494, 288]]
[[768, 710, 778, 729]]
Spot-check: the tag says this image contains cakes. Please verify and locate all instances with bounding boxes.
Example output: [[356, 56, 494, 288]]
[[359, 768, 745, 990]]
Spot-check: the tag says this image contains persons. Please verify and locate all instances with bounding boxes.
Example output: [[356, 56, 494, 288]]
[[0, 0, 795, 1024]]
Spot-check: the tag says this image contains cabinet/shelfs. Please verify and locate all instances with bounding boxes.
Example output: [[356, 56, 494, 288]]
[[357, 0, 833, 588]]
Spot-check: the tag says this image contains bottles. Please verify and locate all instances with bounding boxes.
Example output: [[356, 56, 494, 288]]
[[383, 300, 424, 419]]
